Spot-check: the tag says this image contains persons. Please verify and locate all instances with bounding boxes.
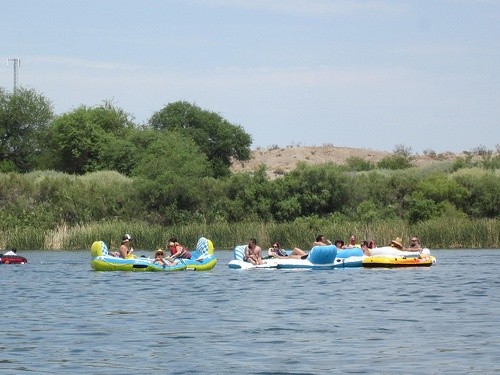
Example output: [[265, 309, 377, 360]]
[[4, 248, 17, 256], [150, 248, 178, 266], [243, 238, 267, 265], [110, 233, 194, 259], [268, 235, 423, 259]]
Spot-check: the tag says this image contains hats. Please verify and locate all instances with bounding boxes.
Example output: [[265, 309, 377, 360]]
[[391, 237, 403, 248], [155, 249, 166, 258], [121, 234, 133, 245]]
